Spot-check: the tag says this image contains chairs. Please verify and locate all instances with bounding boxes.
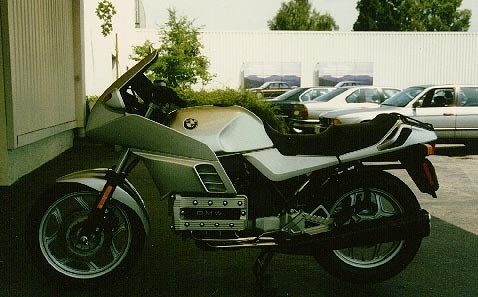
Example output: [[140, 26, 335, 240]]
[[433, 95, 447, 107]]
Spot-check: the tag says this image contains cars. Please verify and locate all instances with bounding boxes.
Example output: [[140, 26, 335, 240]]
[[278, 84, 434, 137], [262, 86, 335, 122], [245, 81, 299, 99], [316, 84, 478, 155]]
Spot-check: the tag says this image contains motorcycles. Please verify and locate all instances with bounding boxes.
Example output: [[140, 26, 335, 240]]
[[22, 51, 439, 285]]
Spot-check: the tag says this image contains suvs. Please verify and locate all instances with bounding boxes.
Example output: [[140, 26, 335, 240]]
[[334, 81, 361, 88]]
